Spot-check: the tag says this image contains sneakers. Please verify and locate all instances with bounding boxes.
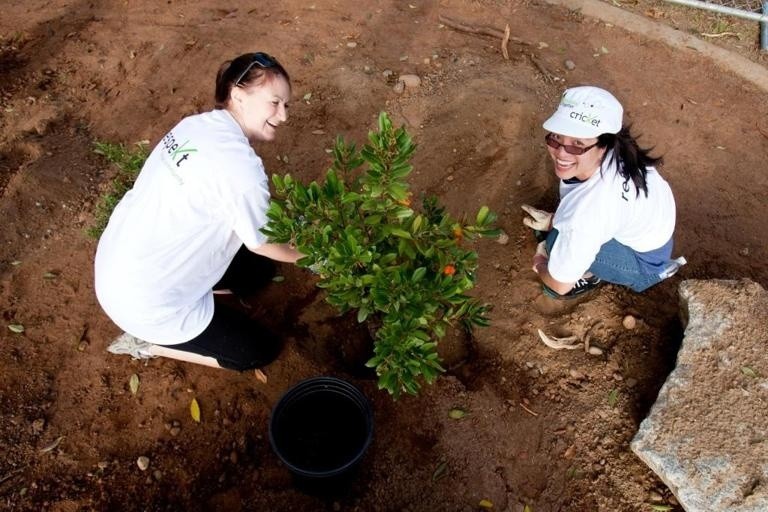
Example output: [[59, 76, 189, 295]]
[[106, 332, 155, 360], [543, 276, 601, 300]]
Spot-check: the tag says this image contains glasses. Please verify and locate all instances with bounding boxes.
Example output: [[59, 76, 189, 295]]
[[545, 132, 600, 155], [236, 52, 280, 85]]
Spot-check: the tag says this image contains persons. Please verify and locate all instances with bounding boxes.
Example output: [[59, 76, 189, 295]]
[[520, 86, 676, 299], [94, 52, 331, 372]]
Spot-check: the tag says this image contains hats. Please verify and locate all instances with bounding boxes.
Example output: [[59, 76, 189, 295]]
[[542, 86, 624, 139]]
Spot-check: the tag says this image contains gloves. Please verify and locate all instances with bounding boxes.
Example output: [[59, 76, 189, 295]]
[[532, 240, 550, 273], [521, 203, 554, 232]]
